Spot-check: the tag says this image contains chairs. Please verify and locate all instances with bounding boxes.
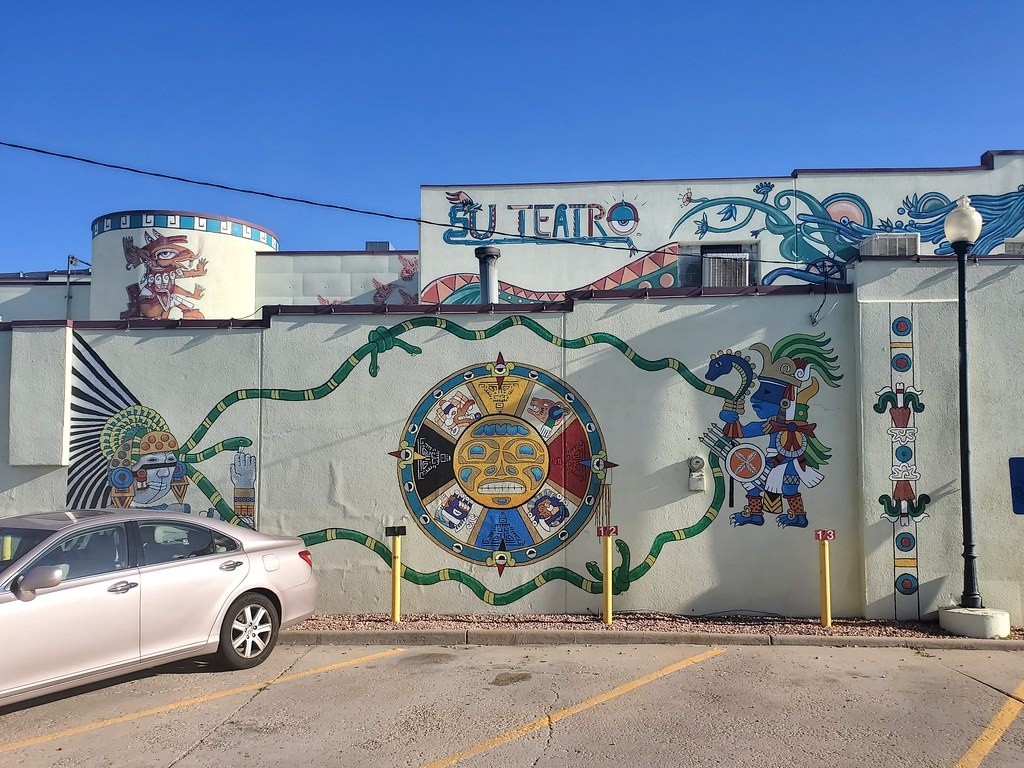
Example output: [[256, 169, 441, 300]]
[[140, 526, 164, 543], [86, 535, 115, 562], [36, 545, 61, 566], [188, 530, 211, 543]]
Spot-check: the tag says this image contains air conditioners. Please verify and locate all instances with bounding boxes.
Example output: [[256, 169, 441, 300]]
[[859, 232, 920, 256], [1004, 238, 1024, 256], [702, 254, 749, 287]]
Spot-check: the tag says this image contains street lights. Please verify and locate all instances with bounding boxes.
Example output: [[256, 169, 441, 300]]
[[942, 193, 984, 614]]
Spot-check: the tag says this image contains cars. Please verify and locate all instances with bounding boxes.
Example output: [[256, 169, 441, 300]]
[[1, 508, 320, 717]]
